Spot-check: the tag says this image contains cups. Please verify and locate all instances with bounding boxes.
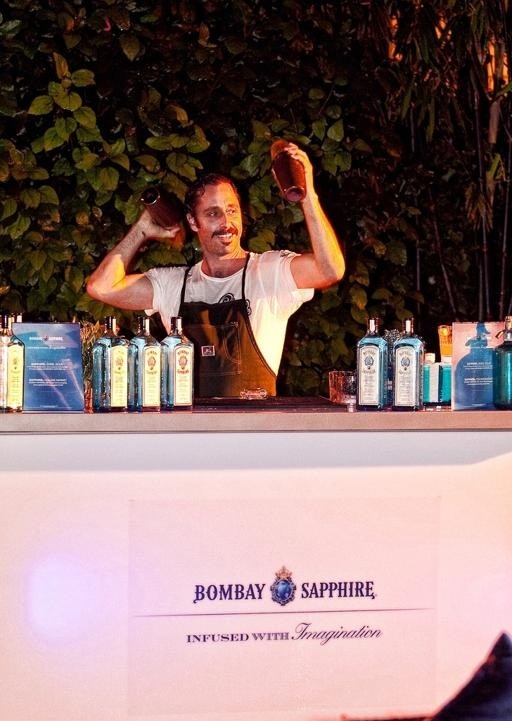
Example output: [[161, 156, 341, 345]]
[[329, 371, 359, 406]]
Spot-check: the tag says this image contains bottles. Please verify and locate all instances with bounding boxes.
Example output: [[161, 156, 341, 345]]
[[92, 316, 195, 412], [1, 313, 25, 413], [268, 141, 307, 203], [141, 186, 192, 242], [358, 315, 512, 411]]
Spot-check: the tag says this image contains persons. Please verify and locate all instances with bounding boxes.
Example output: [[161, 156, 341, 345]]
[[85, 142, 346, 399]]
